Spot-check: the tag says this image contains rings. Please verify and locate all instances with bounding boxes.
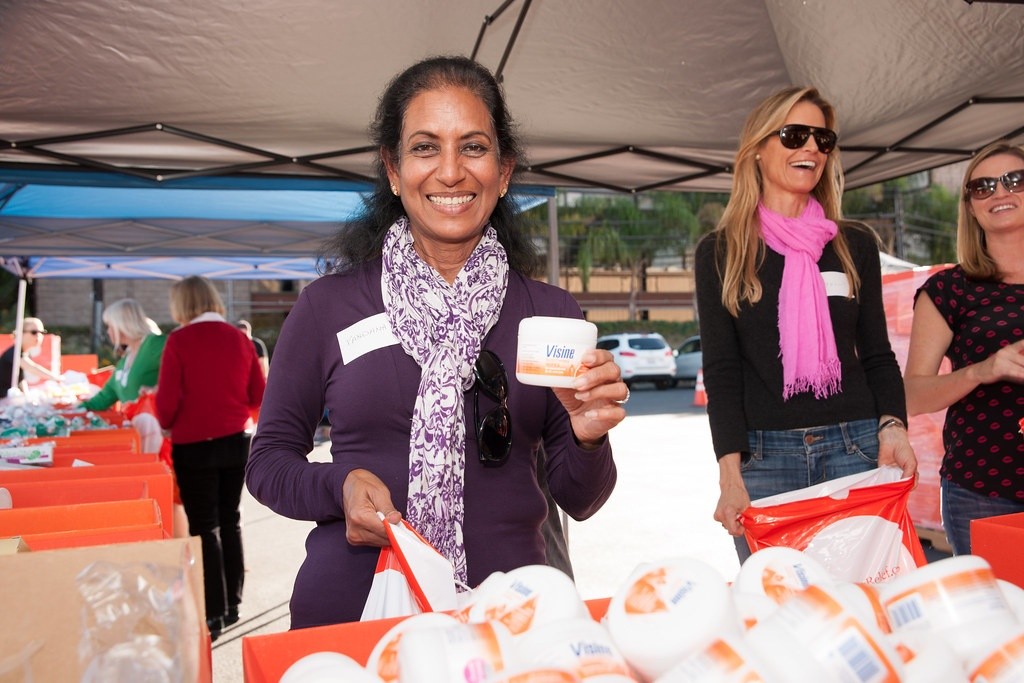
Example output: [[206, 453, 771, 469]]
[[722, 523, 726, 528], [613, 387, 629, 404]]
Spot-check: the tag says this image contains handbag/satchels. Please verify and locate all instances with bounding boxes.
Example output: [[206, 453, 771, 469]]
[[359, 510, 473, 622], [735, 464, 928, 569]]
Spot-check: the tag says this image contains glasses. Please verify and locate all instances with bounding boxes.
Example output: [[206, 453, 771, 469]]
[[772, 124, 837, 154], [965, 169, 1024, 200], [24, 328, 49, 335], [473, 350, 512, 468]]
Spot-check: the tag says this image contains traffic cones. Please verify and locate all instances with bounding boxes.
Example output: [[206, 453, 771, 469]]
[[690, 369, 708, 407]]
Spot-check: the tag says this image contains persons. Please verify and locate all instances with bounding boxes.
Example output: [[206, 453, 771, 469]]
[[237, 318, 269, 380], [153, 277, 269, 642], [68, 297, 169, 413], [903, 140, 1024, 560], [692, 82, 918, 569], [245, 56, 631, 631], [0, 316, 67, 400]]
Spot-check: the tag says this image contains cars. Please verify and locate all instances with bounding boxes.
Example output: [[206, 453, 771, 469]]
[[596, 332, 679, 390], [674, 334, 703, 379]]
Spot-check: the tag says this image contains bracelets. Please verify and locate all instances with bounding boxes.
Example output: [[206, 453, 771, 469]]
[[877, 419, 907, 433]]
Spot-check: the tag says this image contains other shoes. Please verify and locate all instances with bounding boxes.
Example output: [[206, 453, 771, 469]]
[[223, 608, 239, 628], [205, 618, 221, 643]]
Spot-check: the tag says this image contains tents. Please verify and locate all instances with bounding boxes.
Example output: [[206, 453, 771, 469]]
[[0, 173, 562, 396]]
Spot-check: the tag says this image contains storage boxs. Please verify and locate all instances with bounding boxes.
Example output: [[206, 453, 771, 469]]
[[0, 367, 1024, 683]]
